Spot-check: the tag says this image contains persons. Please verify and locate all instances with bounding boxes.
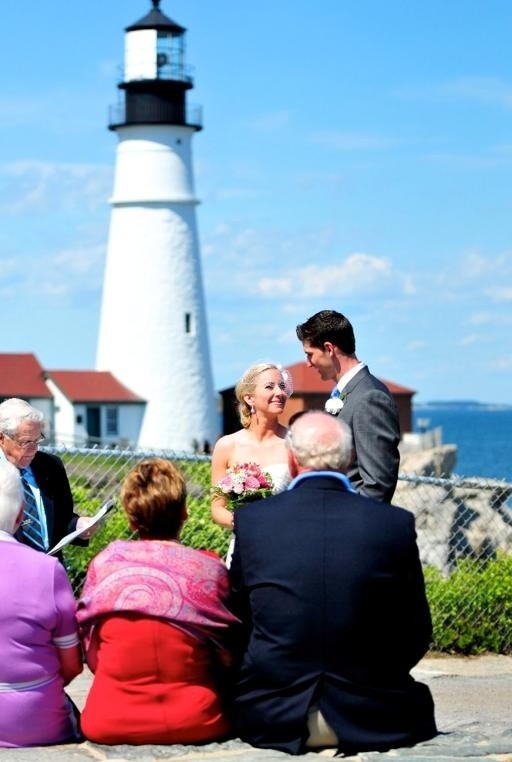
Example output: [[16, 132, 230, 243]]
[[0, 397, 99, 572], [297, 311, 402, 504], [1, 459, 85, 747], [220, 410, 437, 754], [212, 363, 293, 569], [74, 459, 244, 744]]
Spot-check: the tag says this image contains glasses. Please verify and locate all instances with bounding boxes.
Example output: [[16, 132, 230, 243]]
[[4, 431, 45, 447]]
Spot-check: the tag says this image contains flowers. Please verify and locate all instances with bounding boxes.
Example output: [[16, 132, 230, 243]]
[[210, 462, 274, 513]]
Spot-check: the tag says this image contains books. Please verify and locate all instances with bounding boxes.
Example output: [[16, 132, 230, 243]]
[[46, 499, 115, 560]]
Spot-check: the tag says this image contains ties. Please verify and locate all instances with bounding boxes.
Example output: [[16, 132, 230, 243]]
[[18, 469, 46, 553], [331, 389, 340, 396]]
